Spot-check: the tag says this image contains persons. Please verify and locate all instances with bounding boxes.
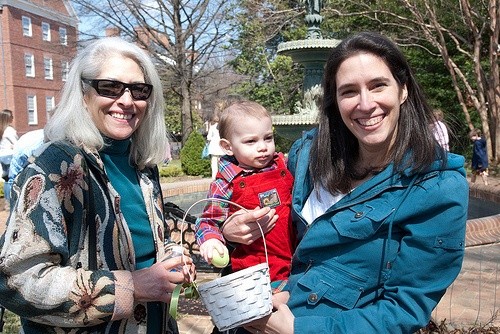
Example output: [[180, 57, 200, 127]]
[[219, 32, 469, 334], [469, 128, 488, 186], [195, 101, 296, 278], [0, 37, 197, 334], [205, 114, 226, 180], [0, 110, 19, 210], [427, 108, 450, 152]]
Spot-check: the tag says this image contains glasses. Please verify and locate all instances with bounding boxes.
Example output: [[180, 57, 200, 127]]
[[81, 77, 153, 100]]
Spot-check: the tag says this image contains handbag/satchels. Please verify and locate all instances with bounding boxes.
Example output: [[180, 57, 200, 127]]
[[202, 141, 211, 159]]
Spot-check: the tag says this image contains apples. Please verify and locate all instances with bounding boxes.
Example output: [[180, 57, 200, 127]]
[[211, 249, 229, 268]]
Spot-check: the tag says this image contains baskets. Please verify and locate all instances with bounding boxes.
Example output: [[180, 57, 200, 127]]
[[180, 198, 273, 332]]
